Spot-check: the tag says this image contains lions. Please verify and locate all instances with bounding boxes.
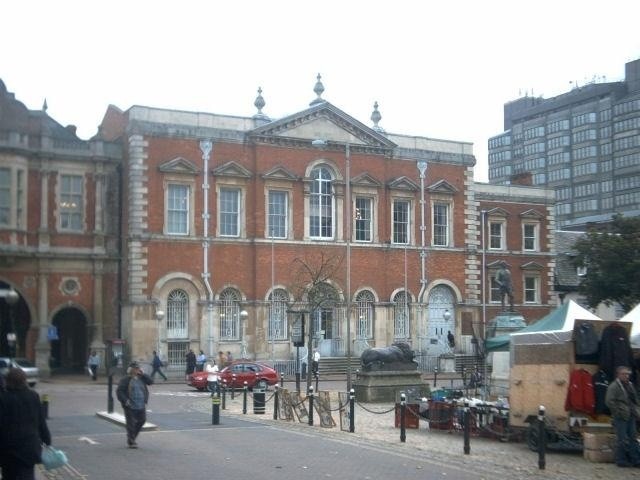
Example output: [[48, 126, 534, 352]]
[[359, 342, 417, 369]]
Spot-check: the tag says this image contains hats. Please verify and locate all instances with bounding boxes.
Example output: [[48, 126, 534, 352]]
[[130, 362, 139, 369]]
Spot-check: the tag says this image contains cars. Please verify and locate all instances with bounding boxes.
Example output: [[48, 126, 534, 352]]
[[184, 360, 283, 391], [0, 356, 41, 387]]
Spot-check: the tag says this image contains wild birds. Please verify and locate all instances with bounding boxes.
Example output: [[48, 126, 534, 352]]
[[448, 328, 456, 345]]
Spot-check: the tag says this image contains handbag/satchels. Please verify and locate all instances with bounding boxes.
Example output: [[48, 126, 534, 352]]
[[40, 444, 68, 470]]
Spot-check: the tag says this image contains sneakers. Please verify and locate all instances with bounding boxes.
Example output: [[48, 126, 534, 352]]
[[127, 438, 138, 448], [618, 462, 640, 468]]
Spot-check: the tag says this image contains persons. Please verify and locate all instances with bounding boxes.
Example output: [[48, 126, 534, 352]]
[[493, 261, 515, 312], [447, 331, 454, 348], [226, 351, 233, 365], [604, 365, 640, 469], [150, 350, 167, 380], [311, 349, 321, 373], [196, 350, 205, 373], [0, 366, 52, 480], [185, 349, 197, 377], [115, 362, 155, 449], [217, 351, 226, 371], [469, 367, 483, 389], [88, 351, 100, 380]]
